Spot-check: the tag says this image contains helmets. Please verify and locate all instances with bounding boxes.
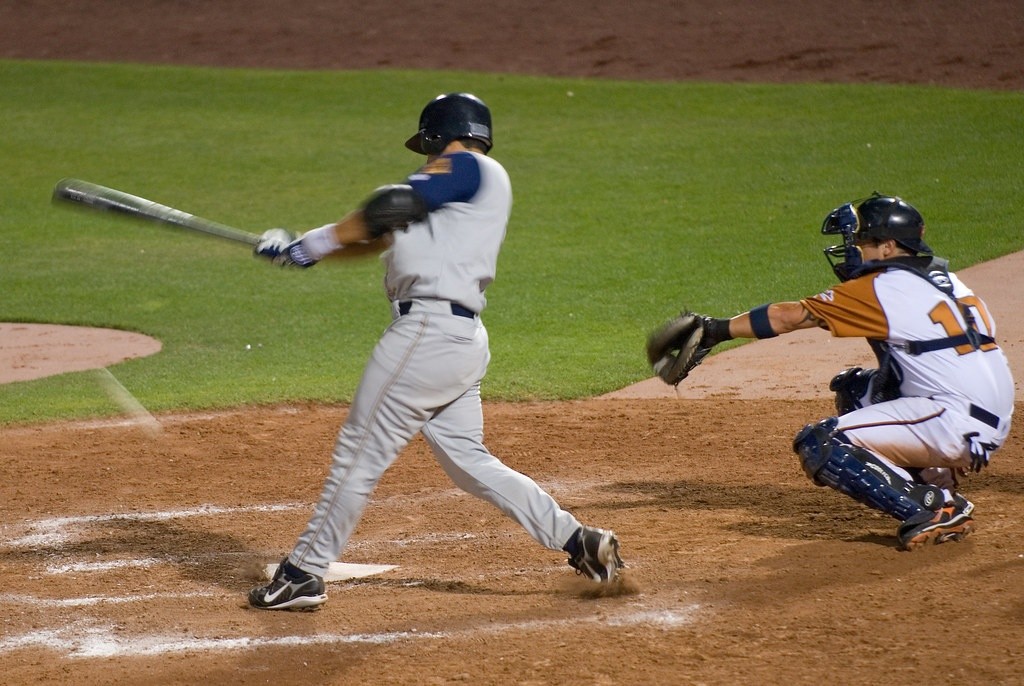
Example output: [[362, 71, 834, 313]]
[[404, 92, 494, 155], [821, 191, 935, 255]]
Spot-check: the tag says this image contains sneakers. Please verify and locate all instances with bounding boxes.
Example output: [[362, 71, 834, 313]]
[[248, 555, 329, 610], [901, 489, 978, 553], [567, 524, 625, 585]]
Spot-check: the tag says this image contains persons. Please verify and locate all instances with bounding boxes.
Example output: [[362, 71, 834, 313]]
[[246, 92, 626, 613], [646, 191, 1014, 552]]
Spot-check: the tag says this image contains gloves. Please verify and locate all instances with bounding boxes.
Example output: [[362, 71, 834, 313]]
[[273, 222, 347, 270], [254, 227, 292, 257]]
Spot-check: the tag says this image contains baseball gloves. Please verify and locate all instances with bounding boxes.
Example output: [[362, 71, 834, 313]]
[[642, 311, 722, 387]]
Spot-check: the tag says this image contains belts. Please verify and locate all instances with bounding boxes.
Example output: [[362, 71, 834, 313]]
[[398, 301, 475, 320]]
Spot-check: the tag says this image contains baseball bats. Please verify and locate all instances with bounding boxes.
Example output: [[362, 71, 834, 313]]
[[49, 175, 264, 246]]
